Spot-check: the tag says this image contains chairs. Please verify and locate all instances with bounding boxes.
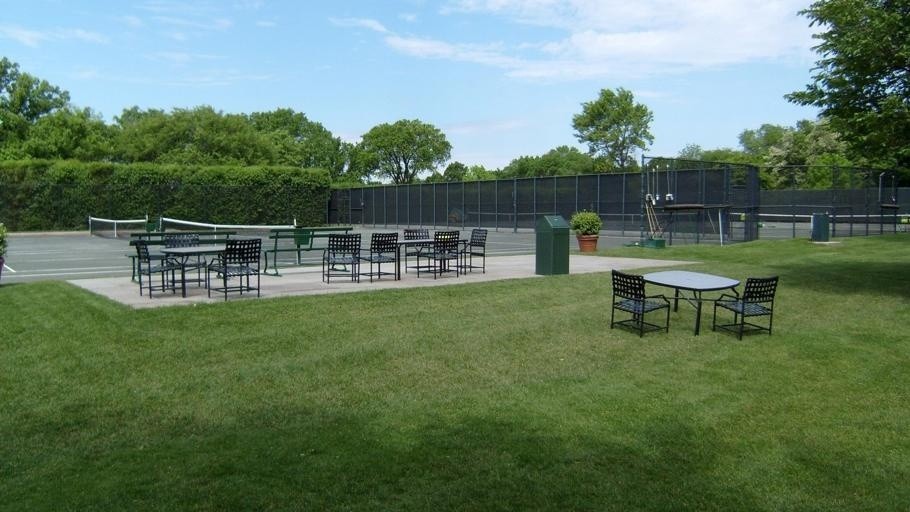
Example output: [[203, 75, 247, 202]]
[[207, 238, 262, 302], [163, 232, 207, 289], [609, 268, 669, 337], [713, 275, 778, 341], [134, 240, 183, 299], [322, 227, 488, 283]]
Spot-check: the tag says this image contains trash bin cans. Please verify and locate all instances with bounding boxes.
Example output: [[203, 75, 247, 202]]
[[535, 215, 570, 275], [812, 213, 829, 242]]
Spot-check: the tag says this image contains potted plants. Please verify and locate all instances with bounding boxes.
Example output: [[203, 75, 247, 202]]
[[567, 208, 603, 253]]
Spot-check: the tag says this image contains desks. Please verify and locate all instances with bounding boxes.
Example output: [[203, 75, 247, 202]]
[[156, 244, 262, 298], [639, 270, 741, 336]]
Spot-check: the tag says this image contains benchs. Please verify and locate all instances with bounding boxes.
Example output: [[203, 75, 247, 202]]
[[262, 226, 353, 276], [128, 230, 238, 285]]
